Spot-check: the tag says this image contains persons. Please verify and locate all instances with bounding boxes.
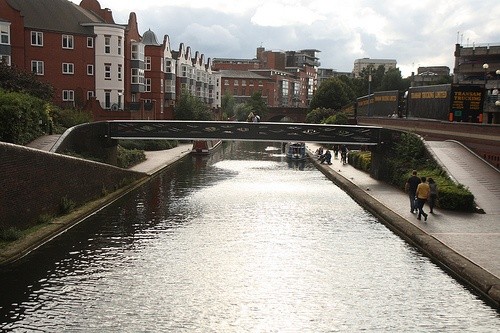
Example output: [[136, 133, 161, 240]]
[[391, 111, 398, 119], [316, 147, 331, 165], [405, 170, 421, 213], [340, 144, 349, 163], [251, 114, 260, 135], [426, 178, 437, 214], [416, 177, 430, 221], [332, 145, 339, 157]]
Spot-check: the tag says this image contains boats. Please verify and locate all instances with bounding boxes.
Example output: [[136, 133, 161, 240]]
[[284, 141, 306, 162], [281, 139, 289, 154], [264, 146, 281, 154]]
